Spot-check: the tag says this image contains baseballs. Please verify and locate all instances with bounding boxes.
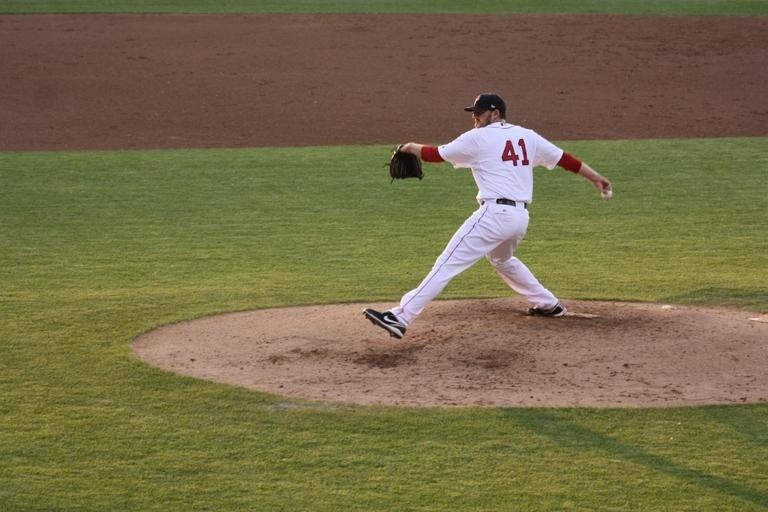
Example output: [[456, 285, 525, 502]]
[[601, 189, 613, 199]]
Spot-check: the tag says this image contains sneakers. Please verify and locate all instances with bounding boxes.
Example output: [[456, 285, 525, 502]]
[[362, 306, 407, 340], [525, 300, 568, 318]]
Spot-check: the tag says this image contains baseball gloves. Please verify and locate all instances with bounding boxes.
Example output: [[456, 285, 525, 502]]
[[389, 145, 423, 179]]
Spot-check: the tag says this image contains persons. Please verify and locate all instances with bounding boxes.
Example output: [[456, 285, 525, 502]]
[[360, 90, 614, 341]]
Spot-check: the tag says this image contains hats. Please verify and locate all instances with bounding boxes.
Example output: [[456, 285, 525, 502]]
[[463, 92, 506, 112]]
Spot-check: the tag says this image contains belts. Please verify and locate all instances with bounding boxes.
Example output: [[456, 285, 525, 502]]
[[480, 198, 528, 210]]
